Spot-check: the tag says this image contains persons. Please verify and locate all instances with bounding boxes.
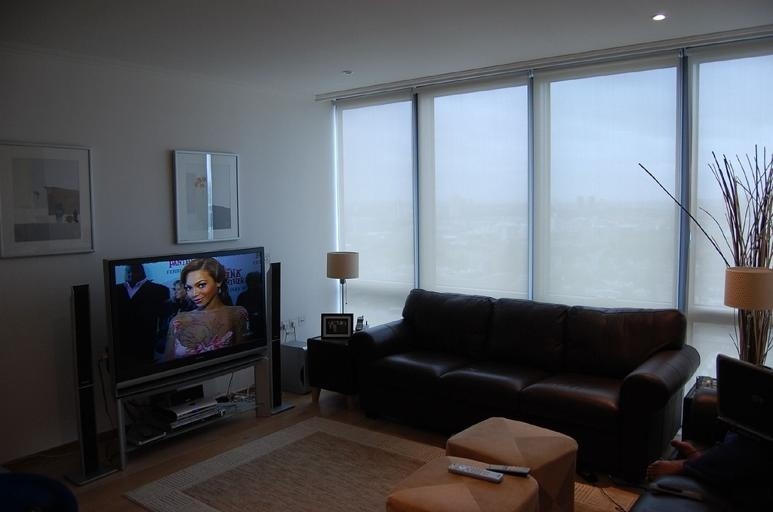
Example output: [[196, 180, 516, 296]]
[[646, 432, 773, 511], [118, 258, 263, 363], [327, 319, 347, 334]]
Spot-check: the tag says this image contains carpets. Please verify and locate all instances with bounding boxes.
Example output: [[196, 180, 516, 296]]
[[121, 412, 636, 511]]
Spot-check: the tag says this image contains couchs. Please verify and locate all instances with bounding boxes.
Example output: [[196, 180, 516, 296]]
[[444, 415, 578, 512], [356, 289, 700, 492], [627, 352, 772, 511], [384, 456, 544, 512]]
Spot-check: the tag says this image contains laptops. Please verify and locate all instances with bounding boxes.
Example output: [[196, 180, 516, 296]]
[[716, 356, 773, 442]]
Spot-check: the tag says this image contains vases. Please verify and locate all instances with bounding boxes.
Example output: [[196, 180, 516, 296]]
[[735, 308, 769, 364]]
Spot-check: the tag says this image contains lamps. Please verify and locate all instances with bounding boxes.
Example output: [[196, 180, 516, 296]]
[[723, 263, 771, 366], [325, 251, 359, 315]]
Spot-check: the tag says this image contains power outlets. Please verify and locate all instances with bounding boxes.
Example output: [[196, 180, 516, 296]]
[[280, 317, 299, 334]]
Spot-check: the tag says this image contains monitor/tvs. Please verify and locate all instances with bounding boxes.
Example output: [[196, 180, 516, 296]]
[[103, 247, 272, 399]]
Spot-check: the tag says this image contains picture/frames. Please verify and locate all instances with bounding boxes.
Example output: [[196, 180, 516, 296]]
[[319, 312, 354, 340], [0, 138, 96, 259], [169, 146, 241, 246]]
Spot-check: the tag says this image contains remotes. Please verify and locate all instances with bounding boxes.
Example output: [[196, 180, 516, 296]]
[[448, 463, 503, 483], [487, 465, 530, 477]]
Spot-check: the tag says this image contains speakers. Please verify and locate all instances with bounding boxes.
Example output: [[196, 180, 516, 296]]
[[266, 262, 295, 415], [65, 285, 119, 486]]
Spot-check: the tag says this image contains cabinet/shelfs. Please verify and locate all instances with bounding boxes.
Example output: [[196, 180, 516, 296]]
[[114, 353, 274, 474], [682, 376, 720, 448], [305, 335, 356, 413], [280, 340, 308, 376]]
